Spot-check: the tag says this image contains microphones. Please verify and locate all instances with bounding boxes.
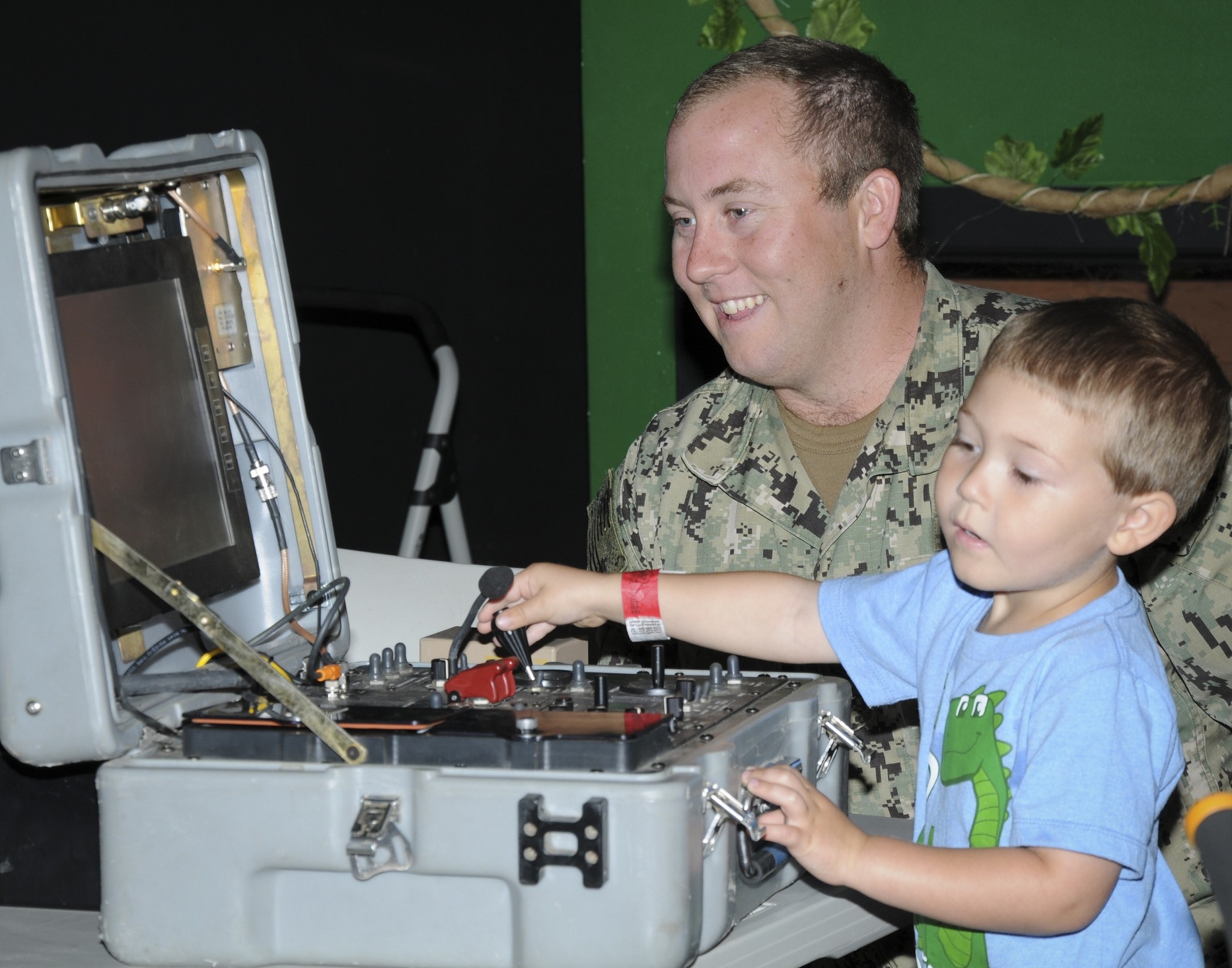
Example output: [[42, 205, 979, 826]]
[[447, 566, 514, 676]]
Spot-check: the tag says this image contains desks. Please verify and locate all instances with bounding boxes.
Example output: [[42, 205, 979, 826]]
[[1, 741, 914, 968]]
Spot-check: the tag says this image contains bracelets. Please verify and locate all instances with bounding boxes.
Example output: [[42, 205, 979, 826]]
[[620, 569, 671, 643]]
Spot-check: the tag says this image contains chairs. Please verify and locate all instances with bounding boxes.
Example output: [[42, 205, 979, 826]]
[[292, 283, 474, 567]]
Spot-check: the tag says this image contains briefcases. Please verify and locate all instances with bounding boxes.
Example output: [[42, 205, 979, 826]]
[[0, 128, 877, 966]]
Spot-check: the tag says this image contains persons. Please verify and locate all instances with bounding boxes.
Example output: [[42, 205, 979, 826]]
[[479, 296, 1231, 968], [579, 35, 1232, 968]]
